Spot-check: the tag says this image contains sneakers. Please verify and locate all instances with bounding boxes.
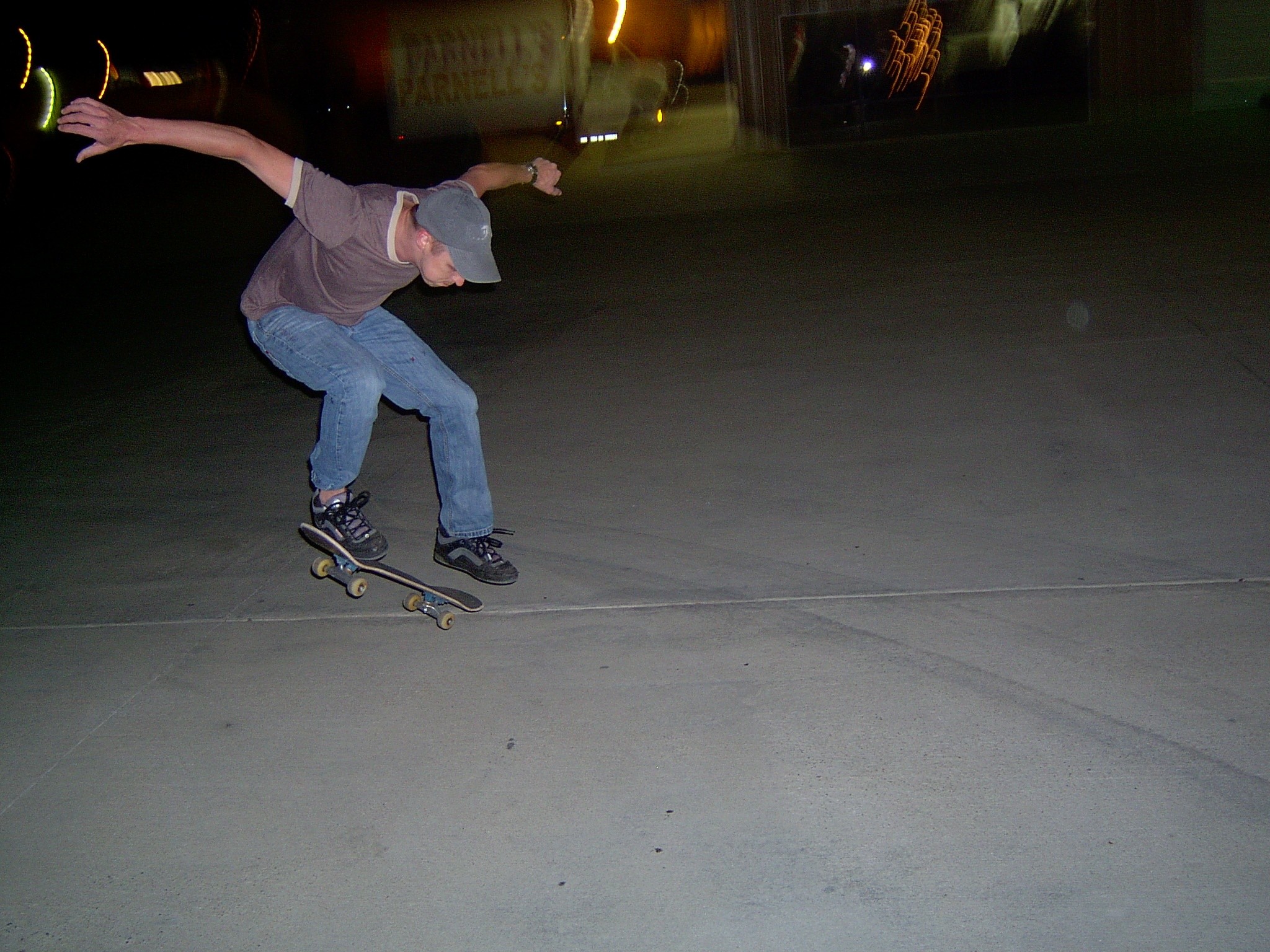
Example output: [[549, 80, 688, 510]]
[[310, 484, 389, 561], [434, 527, 520, 585]]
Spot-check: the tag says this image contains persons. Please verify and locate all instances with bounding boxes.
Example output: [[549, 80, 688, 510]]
[[58, 98, 563, 585]]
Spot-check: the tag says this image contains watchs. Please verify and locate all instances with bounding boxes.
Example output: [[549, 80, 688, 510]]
[[524, 160, 538, 186]]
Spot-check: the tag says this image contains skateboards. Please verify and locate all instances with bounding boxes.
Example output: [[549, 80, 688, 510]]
[[295, 515, 486, 633]]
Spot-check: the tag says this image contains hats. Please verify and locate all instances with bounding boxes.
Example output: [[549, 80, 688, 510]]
[[414, 187, 502, 284]]
